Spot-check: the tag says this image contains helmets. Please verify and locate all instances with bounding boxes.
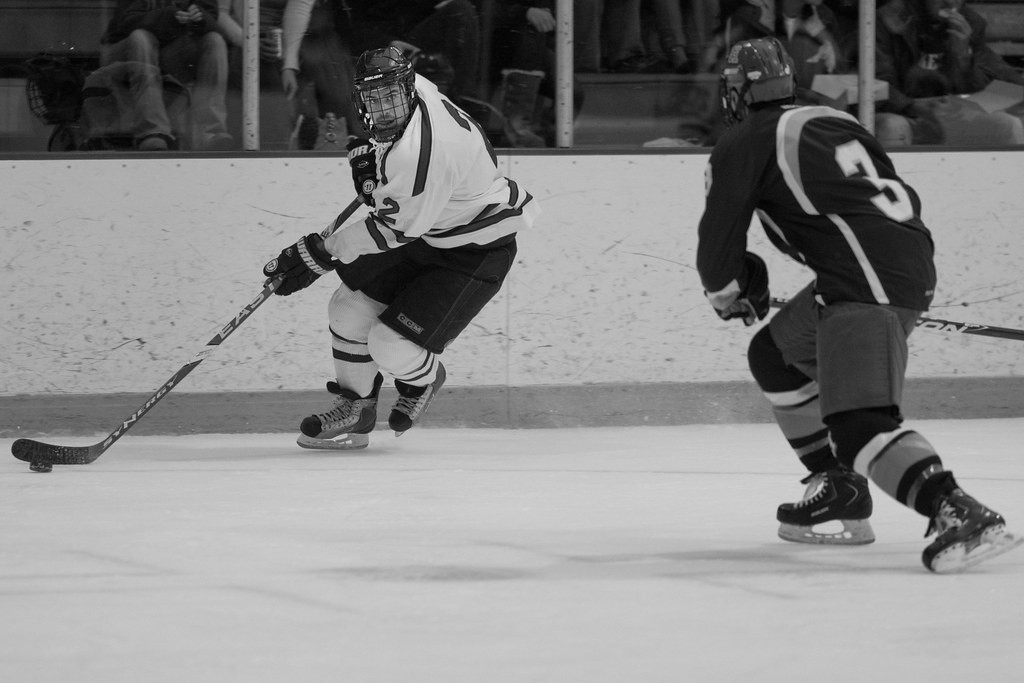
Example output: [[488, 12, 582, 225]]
[[720, 36, 799, 127], [350, 46, 415, 143]]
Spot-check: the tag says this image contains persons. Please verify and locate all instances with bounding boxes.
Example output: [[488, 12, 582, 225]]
[[264, 48, 534, 450], [697, 34, 1024, 574], [0, 0, 1024, 151]]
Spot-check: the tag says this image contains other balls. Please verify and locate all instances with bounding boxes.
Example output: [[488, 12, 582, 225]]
[[29, 459, 53, 473]]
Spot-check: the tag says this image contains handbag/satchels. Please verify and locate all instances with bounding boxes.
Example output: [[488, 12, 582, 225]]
[[289, 112, 348, 150]]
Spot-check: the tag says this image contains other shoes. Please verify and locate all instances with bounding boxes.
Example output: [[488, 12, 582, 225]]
[[139, 136, 167, 150], [192, 131, 235, 151], [609, 60, 657, 73], [671, 47, 690, 73]]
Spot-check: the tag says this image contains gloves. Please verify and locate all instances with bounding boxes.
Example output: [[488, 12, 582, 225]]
[[704, 250, 770, 326], [346, 138, 379, 208], [263, 232, 336, 296]]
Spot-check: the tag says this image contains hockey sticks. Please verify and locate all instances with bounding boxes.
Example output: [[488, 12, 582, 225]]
[[768, 295, 1023, 341], [11, 190, 364, 466]]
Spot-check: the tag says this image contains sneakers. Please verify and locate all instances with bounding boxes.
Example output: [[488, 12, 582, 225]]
[[776, 466, 876, 545], [296, 371, 384, 449], [388, 361, 446, 437], [922, 488, 1024, 574]]
